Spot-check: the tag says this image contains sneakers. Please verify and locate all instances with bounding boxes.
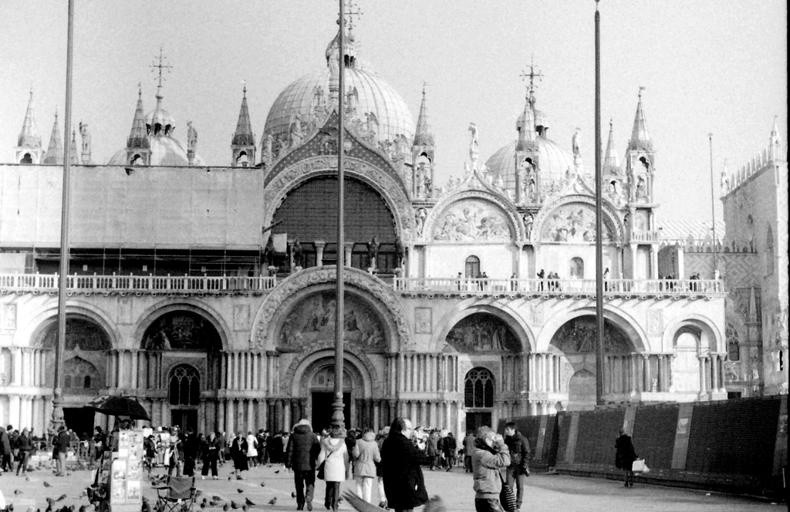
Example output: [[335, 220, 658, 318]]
[[297, 496, 337, 511]]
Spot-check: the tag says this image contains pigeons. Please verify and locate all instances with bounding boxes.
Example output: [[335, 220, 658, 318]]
[[43, 481, 52, 487], [125, 168, 134, 175], [201, 470, 297, 512], [14, 490, 23, 494]]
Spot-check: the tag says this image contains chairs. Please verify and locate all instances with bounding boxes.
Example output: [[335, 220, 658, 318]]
[[152, 475, 200, 512]]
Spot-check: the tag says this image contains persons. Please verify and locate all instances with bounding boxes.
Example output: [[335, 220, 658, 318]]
[[0, 425, 109, 476], [614, 429, 638, 487], [142, 424, 290, 480], [285, 418, 429, 512], [603, 268, 609, 291], [504, 421, 531, 512], [659, 271, 701, 291], [463, 426, 511, 512], [456, 269, 562, 292], [420, 426, 457, 471]]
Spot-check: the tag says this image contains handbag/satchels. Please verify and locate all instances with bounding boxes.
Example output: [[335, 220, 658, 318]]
[[318, 460, 326, 479], [500, 482, 517, 511], [633, 459, 650, 477]]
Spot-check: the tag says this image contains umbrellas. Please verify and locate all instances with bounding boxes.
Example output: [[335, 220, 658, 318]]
[[84, 396, 152, 422]]
